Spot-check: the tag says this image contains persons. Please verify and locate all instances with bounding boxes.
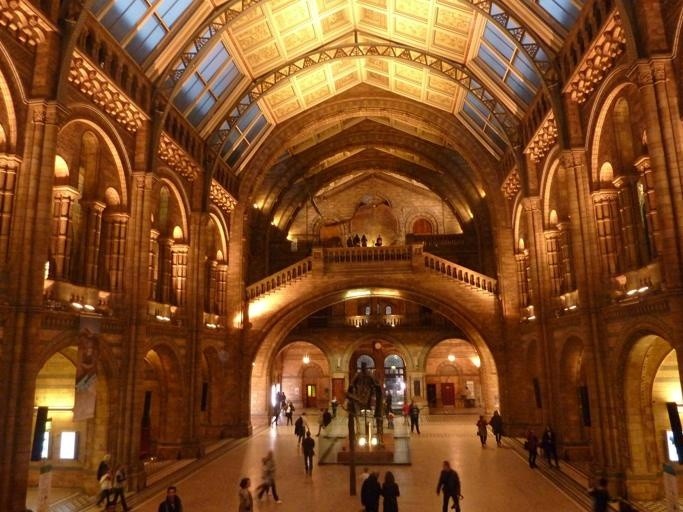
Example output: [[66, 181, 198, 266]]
[[348, 233, 383, 247], [588, 477, 623, 512]]
